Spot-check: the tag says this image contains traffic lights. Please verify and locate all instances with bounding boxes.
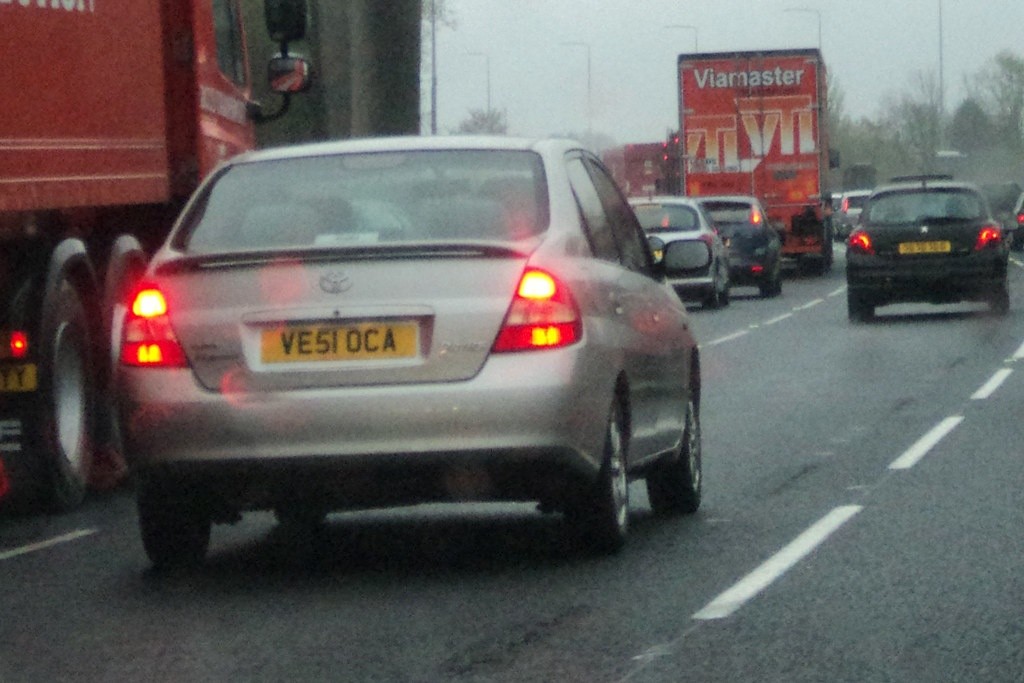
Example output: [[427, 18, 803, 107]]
[[662, 143, 670, 162], [674, 137, 680, 145]]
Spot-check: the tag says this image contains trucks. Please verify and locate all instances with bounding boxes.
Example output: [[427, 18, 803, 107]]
[[678, 46, 842, 274], [599, 142, 677, 197]]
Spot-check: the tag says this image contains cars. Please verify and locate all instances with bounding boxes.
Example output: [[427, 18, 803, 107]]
[[627, 194, 732, 310], [108, 132, 706, 576], [840, 189, 873, 240], [1007, 192, 1024, 254], [832, 193, 844, 242], [692, 195, 783, 298]]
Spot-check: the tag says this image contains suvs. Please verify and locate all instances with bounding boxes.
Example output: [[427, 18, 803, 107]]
[[845, 172, 1011, 321]]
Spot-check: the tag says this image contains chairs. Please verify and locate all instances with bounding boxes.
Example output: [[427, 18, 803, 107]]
[[285, 190, 354, 248], [945, 194, 970, 216], [410, 192, 513, 239], [479, 176, 538, 218]]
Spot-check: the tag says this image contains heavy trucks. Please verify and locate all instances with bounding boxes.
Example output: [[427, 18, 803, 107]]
[[0, 0, 316, 511]]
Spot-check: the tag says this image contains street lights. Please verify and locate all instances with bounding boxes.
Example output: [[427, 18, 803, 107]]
[[560, 38, 593, 109], [665, 22, 700, 55], [781, 6, 823, 48], [461, 51, 493, 112]]
